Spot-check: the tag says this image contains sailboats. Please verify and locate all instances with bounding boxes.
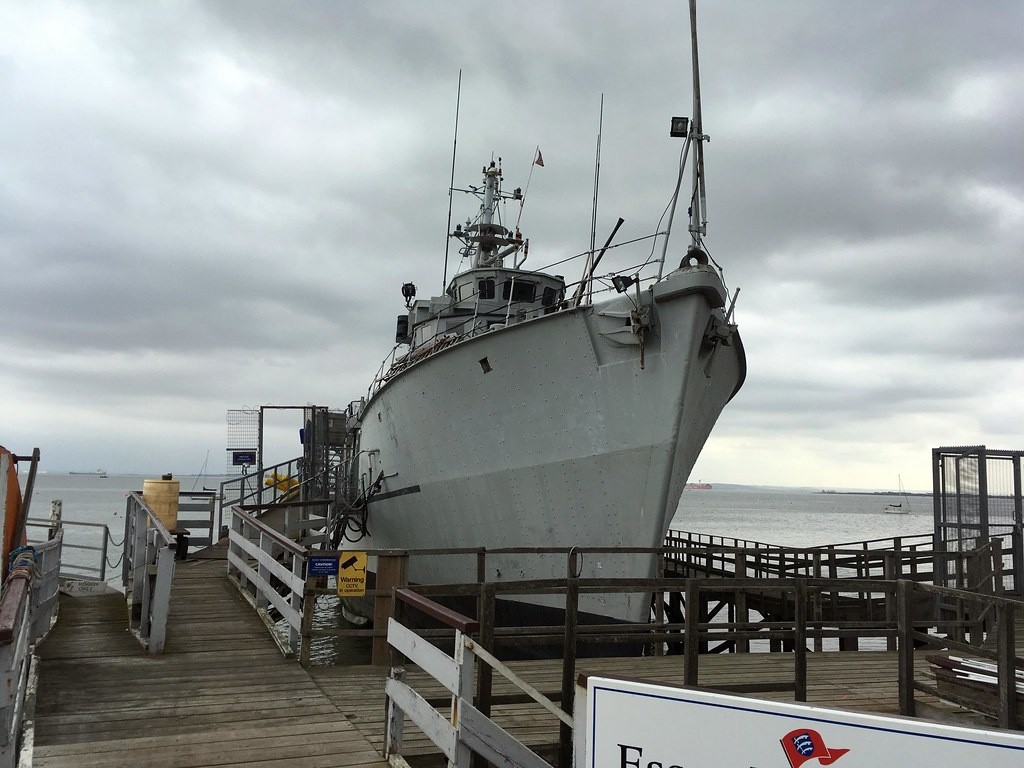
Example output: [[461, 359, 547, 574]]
[[190, 450, 226, 500], [884, 473, 911, 514]]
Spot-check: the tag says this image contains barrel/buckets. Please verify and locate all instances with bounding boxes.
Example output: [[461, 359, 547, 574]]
[[143, 479, 179, 534]]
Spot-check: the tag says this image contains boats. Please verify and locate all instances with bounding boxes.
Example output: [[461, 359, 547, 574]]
[[323, 0, 747, 655]]
